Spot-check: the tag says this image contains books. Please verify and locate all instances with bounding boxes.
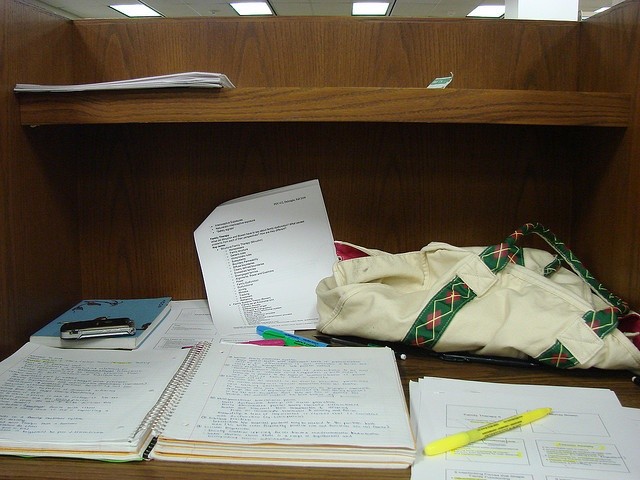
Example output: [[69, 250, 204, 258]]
[[14, 71, 236, 93], [0, 341, 417, 470], [30, 296, 172, 350]]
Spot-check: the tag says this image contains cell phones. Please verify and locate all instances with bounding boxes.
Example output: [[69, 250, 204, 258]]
[[60, 317, 138, 341]]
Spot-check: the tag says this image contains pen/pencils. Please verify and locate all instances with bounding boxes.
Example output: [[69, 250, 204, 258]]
[[424, 407, 552, 456], [632, 377, 640, 387], [260, 330, 311, 346], [257, 326, 333, 346], [440, 354, 541, 367], [183, 339, 285, 350], [313, 333, 406, 360]]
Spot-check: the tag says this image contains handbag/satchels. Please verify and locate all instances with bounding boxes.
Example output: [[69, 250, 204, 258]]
[[315, 221, 640, 372]]
